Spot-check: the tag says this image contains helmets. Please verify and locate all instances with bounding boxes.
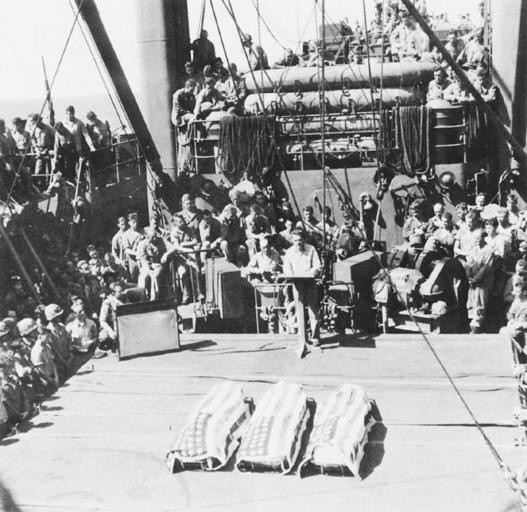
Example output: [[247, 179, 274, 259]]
[[44, 303, 64, 321], [17, 317, 38, 337]]
